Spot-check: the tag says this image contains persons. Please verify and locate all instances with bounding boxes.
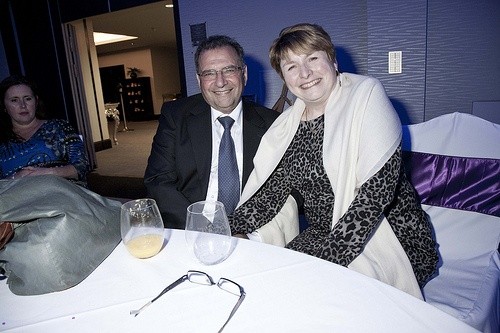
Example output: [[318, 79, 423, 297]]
[[0, 75, 90, 187], [206, 23, 439, 302], [143, 34, 303, 241]]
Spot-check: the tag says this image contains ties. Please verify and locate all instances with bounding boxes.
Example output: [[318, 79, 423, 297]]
[[218, 116, 240, 216]]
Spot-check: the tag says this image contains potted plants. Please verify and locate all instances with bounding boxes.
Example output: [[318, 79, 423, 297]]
[[126, 65, 142, 78]]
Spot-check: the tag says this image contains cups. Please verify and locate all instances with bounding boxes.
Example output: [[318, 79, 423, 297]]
[[185, 201, 233, 265], [119, 198, 165, 260]]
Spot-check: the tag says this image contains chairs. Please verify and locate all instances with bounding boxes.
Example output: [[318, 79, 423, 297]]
[[402, 111, 500, 333]]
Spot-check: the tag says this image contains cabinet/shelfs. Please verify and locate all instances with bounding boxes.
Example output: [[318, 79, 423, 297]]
[[123, 77, 155, 122]]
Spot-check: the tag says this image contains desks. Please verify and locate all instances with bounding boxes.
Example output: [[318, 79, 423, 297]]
[[0, 225, 483, 333], [104, 103, 121, 145]]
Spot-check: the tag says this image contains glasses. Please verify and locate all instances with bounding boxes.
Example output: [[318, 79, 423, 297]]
[[130, 270, 246, 333], [198, 66, 241, 81]]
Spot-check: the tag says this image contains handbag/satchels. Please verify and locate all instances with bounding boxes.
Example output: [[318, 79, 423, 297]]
[[1, 173, 129, 295]]
